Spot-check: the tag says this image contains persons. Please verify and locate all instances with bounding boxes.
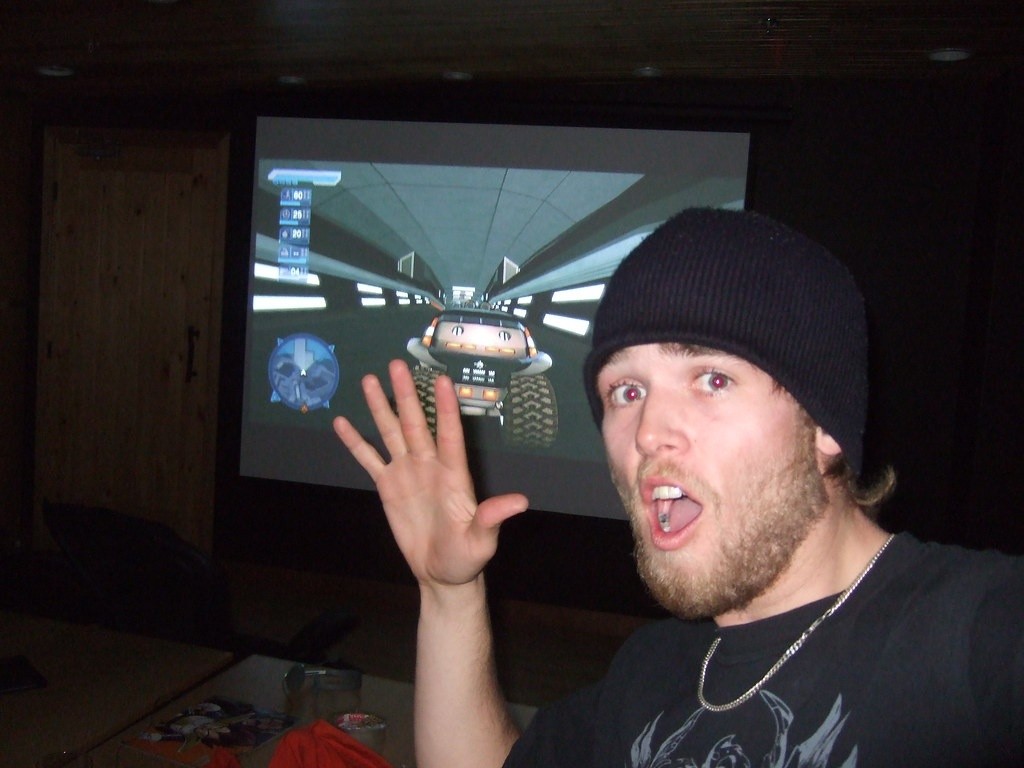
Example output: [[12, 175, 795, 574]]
[[333, 206, 1024, 768]]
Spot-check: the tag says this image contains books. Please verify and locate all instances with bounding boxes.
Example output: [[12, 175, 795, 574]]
[[122, 694, 299, 768]]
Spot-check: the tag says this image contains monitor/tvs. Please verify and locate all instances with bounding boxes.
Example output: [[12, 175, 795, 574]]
[[214, 89, 802, 622]]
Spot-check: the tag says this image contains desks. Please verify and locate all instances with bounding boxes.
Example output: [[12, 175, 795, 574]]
[[52, 652, 536, 768], [0, 609, 234, 766]]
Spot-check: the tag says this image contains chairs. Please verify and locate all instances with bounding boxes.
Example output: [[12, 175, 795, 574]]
[[39, 499, 350, 666]]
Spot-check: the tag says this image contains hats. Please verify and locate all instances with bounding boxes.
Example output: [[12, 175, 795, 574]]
[[583, 205, 867, 479]]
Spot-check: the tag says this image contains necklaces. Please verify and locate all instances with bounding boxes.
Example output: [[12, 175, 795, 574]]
[[698, 534, 895, 711]]
